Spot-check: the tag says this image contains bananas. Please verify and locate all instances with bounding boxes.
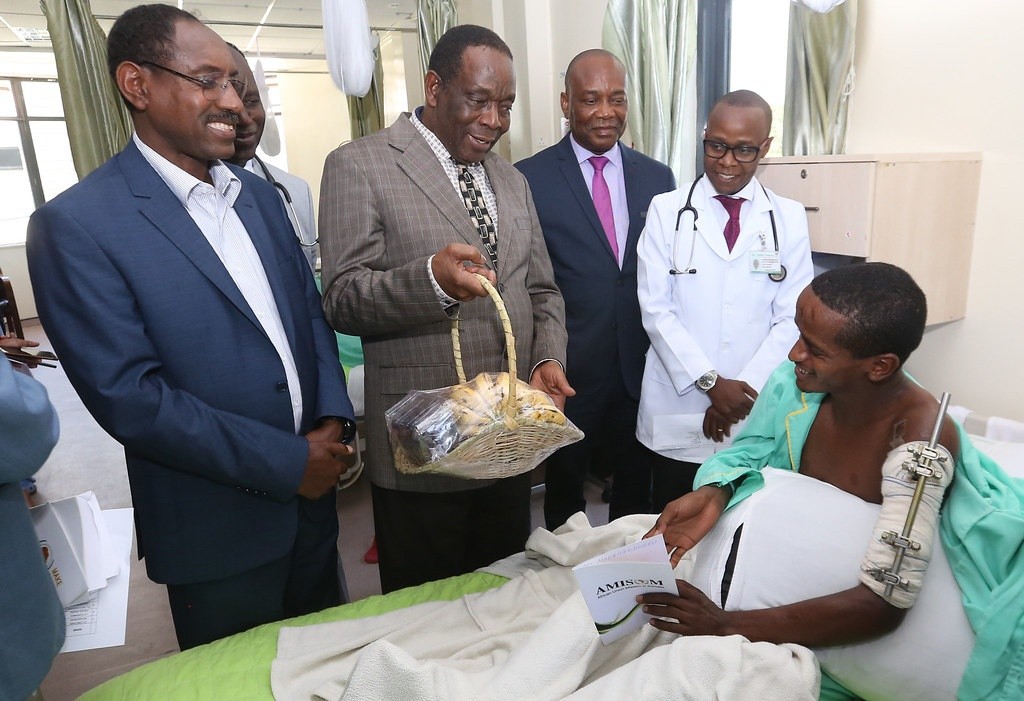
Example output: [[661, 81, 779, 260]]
[[443, 371, 567, 442]]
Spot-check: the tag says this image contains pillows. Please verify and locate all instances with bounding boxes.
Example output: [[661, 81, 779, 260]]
[[686, 465, 975, 701]]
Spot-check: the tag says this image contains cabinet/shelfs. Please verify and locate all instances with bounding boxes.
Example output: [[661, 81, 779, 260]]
[[755, 150, 982, 327]]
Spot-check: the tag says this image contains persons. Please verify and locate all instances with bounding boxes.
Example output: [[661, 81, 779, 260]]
[[317, 23, 577, 596], [511, 47, 676, 534], [633, 265, 961, 652], [0, 332, 60, 701], [635, 88, 814, 513], [24, 3, 364, 651]]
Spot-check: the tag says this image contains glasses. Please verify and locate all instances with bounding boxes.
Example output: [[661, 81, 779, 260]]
[[139, 60, 246, 101], [703, 139, 760, 163]]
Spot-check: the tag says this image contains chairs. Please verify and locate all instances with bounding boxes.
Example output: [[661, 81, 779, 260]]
[[0, 268, 25, 340]]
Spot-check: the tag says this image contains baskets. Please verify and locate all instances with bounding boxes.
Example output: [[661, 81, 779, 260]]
[[385, 275, 585, 479]]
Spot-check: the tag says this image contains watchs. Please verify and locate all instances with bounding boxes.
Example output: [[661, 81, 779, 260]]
[[695, 370, 719, 393]]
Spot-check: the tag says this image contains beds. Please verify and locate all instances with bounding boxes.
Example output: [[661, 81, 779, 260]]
[[78, 405, 1024, 701]]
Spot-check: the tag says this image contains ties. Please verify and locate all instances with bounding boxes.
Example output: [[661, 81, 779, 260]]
[[587, 156, 619, 272], [451, 157, 497, 273], [714, 195, 747, 256]]
